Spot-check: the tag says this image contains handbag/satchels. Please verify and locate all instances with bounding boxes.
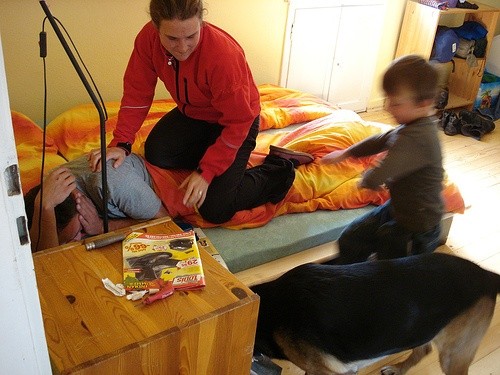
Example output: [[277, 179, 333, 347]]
[[431, 21, 488, 63]]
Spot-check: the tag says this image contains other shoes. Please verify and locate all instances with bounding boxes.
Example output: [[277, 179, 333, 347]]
[[269, 145, 314, 166]]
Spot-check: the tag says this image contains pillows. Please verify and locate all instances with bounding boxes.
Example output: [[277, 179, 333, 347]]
[[10, 108, 69, 200]]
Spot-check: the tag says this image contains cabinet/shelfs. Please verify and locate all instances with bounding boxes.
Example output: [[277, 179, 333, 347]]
[[393, 0, 500, 121]]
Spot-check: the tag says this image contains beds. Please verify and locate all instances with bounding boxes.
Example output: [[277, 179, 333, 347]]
[[44, 88, 453, 284]]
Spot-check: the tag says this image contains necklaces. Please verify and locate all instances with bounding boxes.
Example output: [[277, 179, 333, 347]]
[[158, 43, 174, 66]]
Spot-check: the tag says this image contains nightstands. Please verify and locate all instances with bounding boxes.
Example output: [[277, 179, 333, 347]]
[[29, 214, 260, 375]]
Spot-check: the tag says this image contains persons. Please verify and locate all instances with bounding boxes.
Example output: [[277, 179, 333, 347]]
[[88, 0, 315, 225], [23, 151, 175, 253], [319, 54, 445, 265]]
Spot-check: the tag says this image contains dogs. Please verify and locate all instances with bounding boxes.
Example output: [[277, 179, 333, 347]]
[[248, 253, 500, 375]]
